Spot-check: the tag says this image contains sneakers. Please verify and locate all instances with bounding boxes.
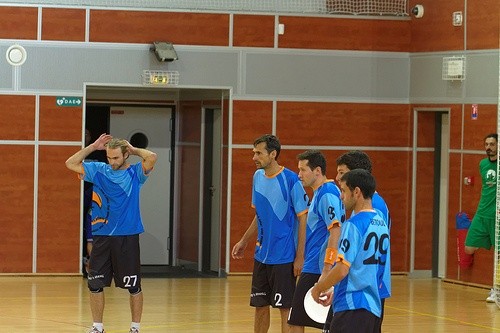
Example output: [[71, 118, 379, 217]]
[[128, 326, 140, 333], [89, 326, 106, 333], [486, 289, 497, 303]]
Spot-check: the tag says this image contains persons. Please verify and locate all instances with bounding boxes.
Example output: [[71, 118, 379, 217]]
[[322, 150, 392, 333], [311, 169, 390, 333], [287, 150, 345, 333], [231, 134, 310, 333], [84, 126, 96, 276], [462, 132, 500, 303], [66, 131, 157, 333]]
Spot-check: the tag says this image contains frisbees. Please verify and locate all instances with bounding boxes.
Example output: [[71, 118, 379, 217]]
[[303, 285, 334, 324]]
